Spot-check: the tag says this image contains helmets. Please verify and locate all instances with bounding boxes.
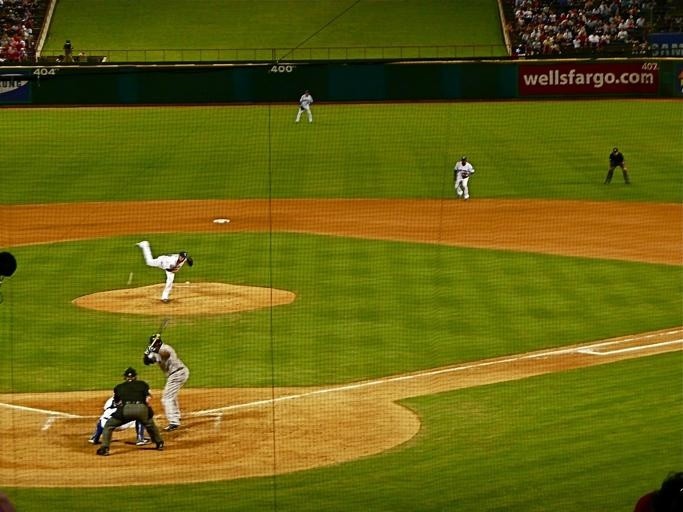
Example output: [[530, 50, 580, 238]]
[[149, 334, 162, 346], [124, 367, 136, 376]]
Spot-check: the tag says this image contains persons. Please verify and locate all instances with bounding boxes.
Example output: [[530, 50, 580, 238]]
[[294, 90, 313, 123], [603, 148, 630, 185], [0, 0, 49, 65], [134, 239, 186, 302], [143, 333, 189, 431], [87, 393, 152, 446], [96, 365, 164, 456], [453, 156, 474, 202], [633, 470, 683, 511], [501, 1, 682, 60], [62, 40, 75, 63]]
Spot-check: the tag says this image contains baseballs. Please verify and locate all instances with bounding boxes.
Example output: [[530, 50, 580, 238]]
[[184, 281, 190, 285]]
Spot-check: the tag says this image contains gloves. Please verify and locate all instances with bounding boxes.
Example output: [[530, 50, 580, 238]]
[[143, 350, 149, 355], [148, 346, 155, 352]]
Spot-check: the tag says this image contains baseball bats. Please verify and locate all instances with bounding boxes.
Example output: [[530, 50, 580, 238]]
[[146, 317, 170, 353]]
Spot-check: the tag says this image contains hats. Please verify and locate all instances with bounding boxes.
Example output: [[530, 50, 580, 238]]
[[462, 156, 466, 160], [180, 252, 186, 257]]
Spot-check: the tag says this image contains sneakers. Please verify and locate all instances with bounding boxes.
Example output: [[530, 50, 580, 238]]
[[88, 438, 98, 443], [155, 441, 164, 449], [96, 447, 109, 455], [163, 424, 178, 430], [136, 438, 150, 446]]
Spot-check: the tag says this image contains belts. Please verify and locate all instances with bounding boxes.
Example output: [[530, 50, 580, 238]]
[[169, 367, 184, 376], [124, 401, 145, 404]]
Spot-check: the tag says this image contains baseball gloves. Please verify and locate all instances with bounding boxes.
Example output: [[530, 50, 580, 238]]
[[461, 172, 468, 179], [186, 256, 194, 266]]
